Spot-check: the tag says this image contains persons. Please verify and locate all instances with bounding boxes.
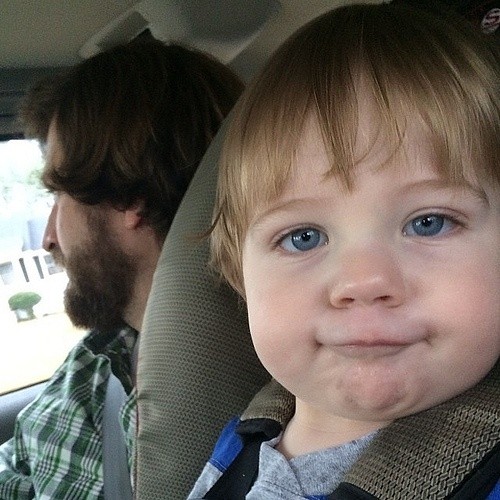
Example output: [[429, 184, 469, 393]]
[[1, 40, 247, 500], [180, 3, 500, 499]]
